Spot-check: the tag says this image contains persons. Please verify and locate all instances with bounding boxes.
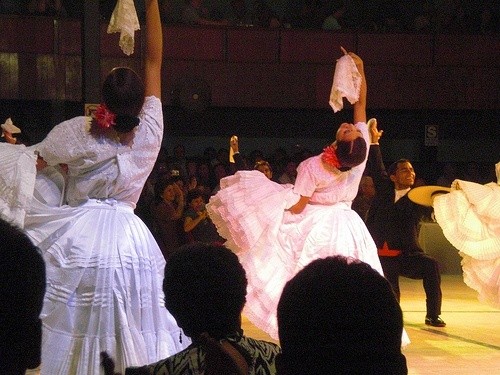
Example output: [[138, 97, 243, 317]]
[[0, 0, 500, 375], [204, 45, 386, 347], [0, 0, 193, 375]]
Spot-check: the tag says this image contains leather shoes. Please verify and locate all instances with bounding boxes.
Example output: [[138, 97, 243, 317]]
[[425, 315, 446, 327]]
